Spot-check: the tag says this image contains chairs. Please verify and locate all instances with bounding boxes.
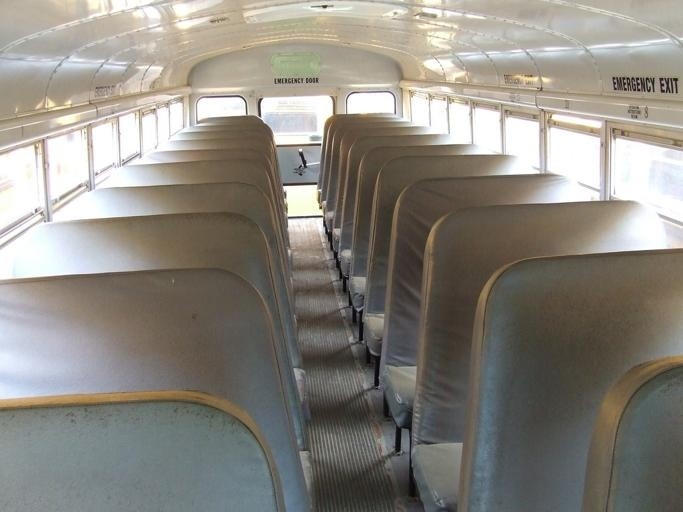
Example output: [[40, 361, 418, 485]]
[[86, 113, 301, 323], [348, 144, 490, 342], [581, 355, 681, 506], [457, 247, 679, 508], [1, 389, 280, 505], [364, 155, 530, 386], [407, 200, 672, 507], [338, 136, 453, 295], [31, 210, 313, 475], [317, 112, 431, 252], [379, 176, 575, 454], [0, 266, 329, 499]]
